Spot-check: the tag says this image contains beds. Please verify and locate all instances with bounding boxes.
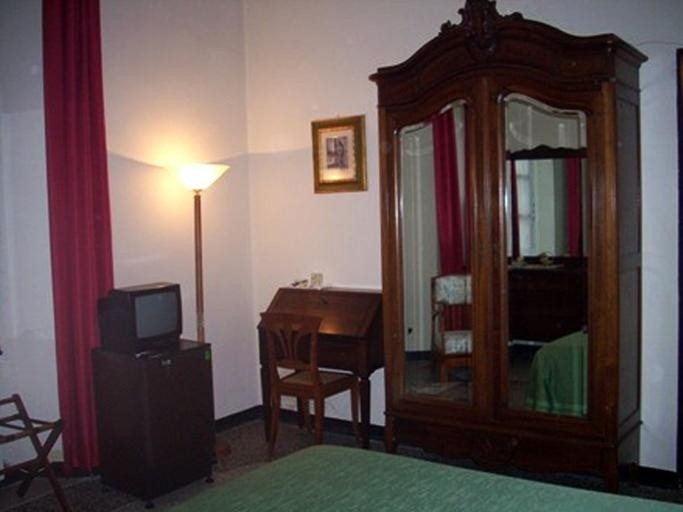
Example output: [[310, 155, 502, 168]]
[[523, 328, 587, 416], [168, 445, 682, 511]]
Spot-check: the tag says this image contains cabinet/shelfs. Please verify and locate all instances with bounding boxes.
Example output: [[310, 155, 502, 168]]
[[91, 339, 215, 511], [367, 2, 649, 492]]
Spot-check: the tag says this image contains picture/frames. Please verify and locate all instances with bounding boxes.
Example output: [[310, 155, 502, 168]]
[[310, 113, 367, 195]]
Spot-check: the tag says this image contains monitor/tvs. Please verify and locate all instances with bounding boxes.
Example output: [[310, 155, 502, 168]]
[[97, 281, 183, 358]]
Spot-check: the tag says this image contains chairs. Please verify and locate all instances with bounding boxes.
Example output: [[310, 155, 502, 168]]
[[258, 311, 359, 462], [427, 270, 472, 387]]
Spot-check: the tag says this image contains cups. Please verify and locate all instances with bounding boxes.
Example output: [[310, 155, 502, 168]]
[[311, 273, 323, 291]]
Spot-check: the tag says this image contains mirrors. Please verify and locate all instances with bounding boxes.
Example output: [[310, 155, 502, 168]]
[[392, 91, 590, 420], [507, 146, 588, 265]]
